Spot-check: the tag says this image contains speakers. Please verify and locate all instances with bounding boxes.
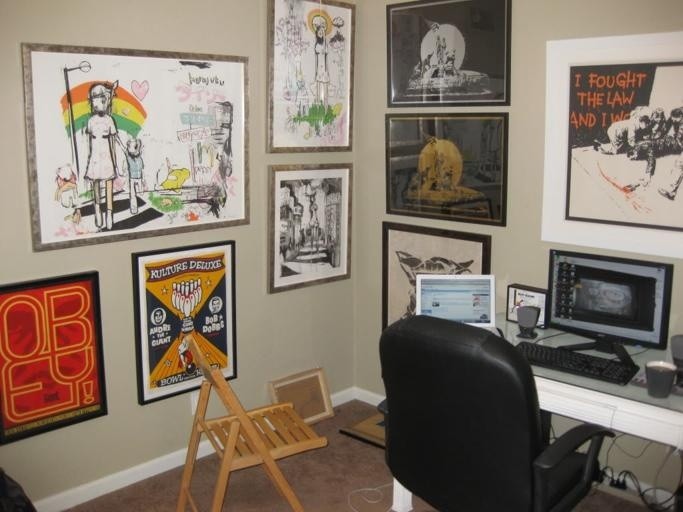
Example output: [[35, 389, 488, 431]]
[[516, 306, 540, 339], [671, 335, 683, 386]]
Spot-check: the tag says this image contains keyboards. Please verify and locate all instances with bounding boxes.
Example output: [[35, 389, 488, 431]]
[[515, 341, 640, 387]]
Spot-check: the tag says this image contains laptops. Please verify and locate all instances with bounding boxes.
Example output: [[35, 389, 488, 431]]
[[415, 273, 502, 340]]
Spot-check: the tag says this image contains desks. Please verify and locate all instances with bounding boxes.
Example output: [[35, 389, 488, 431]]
[[390, 329, 682, 512]]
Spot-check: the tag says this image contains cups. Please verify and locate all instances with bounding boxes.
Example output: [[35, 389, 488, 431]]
[[645, 361, 677, 399]]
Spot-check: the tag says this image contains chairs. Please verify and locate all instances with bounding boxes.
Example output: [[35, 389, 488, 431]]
[[177, 334, 328, 512], [379, 315, 615, 512]]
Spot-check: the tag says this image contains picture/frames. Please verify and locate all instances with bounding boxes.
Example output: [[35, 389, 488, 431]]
[[506, 284, 549, 329], [268, 163, 353, 294], [541, 30, 683, 261], [382, 222, 491, 330], [21, 42, 251, 253], [384, 113, 508, 227], [266, 0, 356, 153], [0, 271, 109, 445], [386, 0, 512, 107], [132, 240, 237, 406], [269, 367, 335, 426]]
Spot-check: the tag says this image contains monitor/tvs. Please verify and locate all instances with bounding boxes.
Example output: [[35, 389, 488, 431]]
[[544, 249, 673, 366]]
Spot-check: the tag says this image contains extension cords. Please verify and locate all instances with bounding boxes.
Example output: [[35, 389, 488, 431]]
[[593, 469, 656, 508]]
